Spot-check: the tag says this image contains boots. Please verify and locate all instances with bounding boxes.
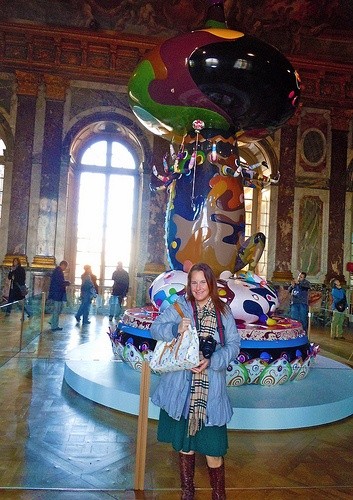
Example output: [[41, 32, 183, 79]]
[[178, 452, 195, 500], [207, 456, 227, 500]]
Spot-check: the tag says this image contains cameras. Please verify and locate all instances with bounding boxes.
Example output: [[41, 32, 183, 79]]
[[197, 335, 218, 359]]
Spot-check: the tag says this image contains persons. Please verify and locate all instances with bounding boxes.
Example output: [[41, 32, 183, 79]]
[[150, 264, 241, 500], [330, 278, 348, 340], [109, 261, 129, 321], [4, 258, 33, 318], [75, 265, 97, 324], [48, 261, 71, 331], [287, 272, 310, 334]]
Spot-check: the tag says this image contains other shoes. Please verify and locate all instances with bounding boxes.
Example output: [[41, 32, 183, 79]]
[[82, 319, 91, 323], [330, 336, 338, 340], [338, 336, 345, 340], [108, 316, 113, 321], [49, 322, 63, 331], [74, 315, 81, 322]]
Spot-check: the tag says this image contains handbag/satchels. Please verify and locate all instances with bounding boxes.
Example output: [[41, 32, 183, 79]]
[[150, 302, 201, 374], [335, 296, 347, 313], [19, 284, 30, 297]]
